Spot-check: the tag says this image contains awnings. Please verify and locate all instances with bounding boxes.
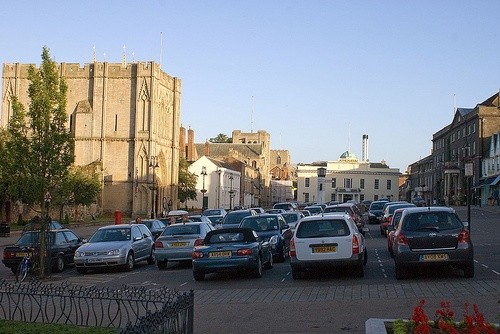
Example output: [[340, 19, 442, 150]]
[[472, 174, 500, 188]]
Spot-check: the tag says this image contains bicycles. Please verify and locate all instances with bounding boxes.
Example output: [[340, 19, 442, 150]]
[[16, 245, 42, 283]]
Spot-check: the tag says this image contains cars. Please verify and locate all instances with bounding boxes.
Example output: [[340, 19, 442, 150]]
[[234, 199, 372, 237], [239, 213, 293, 262], [386, 208, 403, 258], [131, 219, 167, 240], [380, 202, 410, 236], [73, 224, 157, 274], [20, 221, 66, 240], [386, 206, 476, 278], [382, 204, 419, 239], [155, 221, 225, 269], [161, 207, 227, 228], [413, 198, 425, 203], [2, 228, 85, 274], [368, 200, 390, 224]]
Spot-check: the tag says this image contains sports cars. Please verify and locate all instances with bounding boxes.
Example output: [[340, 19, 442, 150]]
[[192, 228, 273, 281]]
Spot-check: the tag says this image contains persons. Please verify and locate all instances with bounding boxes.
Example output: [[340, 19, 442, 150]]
[[170, 217, 176, 224], [135, 216, 141, 223], [182, 213, 192, 223]]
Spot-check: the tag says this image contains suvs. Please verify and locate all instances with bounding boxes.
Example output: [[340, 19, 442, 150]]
[[291, 213, 368, 279], [220, 209, 258, 230]]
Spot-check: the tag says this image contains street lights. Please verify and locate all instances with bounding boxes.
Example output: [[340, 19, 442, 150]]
[[149, 155, 160, 219], [228, 174, 235, 210], [201, 169, 208, 212], [216, 166, 222, 208]]
